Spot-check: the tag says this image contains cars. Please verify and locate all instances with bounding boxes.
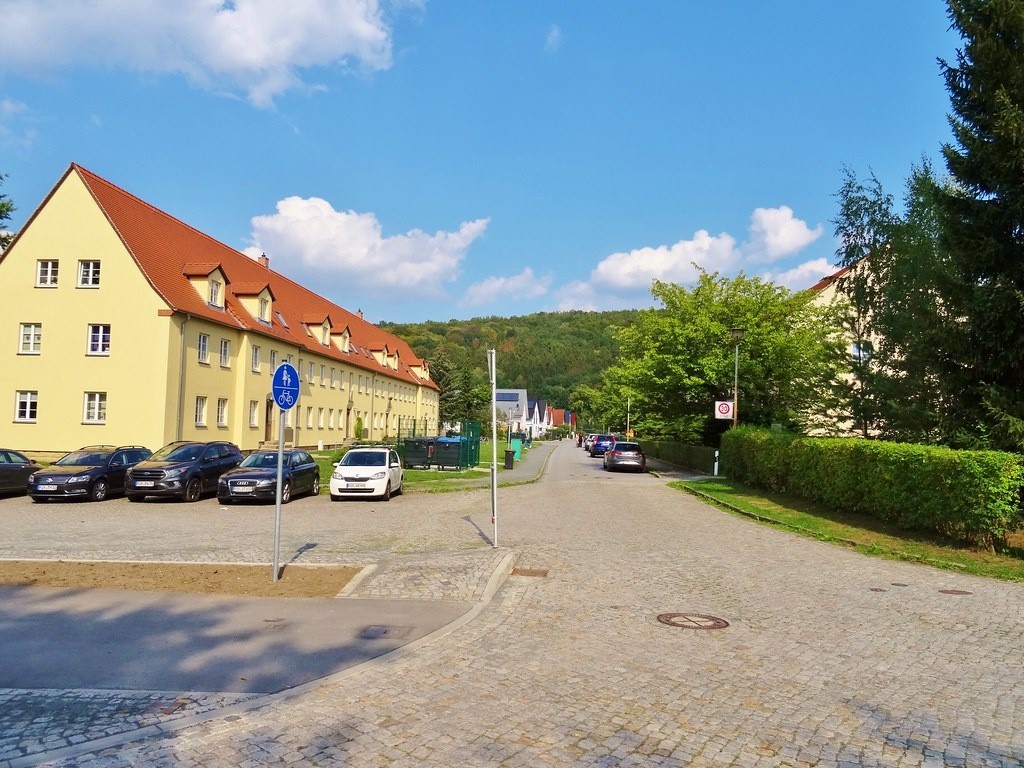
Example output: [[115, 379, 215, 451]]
[[584, 433, 589, 442], [216, 450, 320, 504], [602, 442, 646, 472], [329, 445, 404, 501], [0, 449, 43, 496], [585, 434, 599, 451], [590, 435, 615, 457]]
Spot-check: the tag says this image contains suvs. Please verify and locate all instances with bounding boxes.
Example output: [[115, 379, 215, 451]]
[[124, 440, 243, 503], [27, 445, 153, 503]]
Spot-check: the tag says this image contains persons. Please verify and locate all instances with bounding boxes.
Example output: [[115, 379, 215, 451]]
[[523, 428, 532, 449]]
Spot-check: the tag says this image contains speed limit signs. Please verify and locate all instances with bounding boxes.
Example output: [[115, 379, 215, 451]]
[[714, 401, 732, 419]]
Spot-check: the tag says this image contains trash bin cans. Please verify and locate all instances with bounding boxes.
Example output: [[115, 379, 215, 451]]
[[503, 449, 516, 469], [403, 435, 443, 469], [435, 434, 480, 471]]
